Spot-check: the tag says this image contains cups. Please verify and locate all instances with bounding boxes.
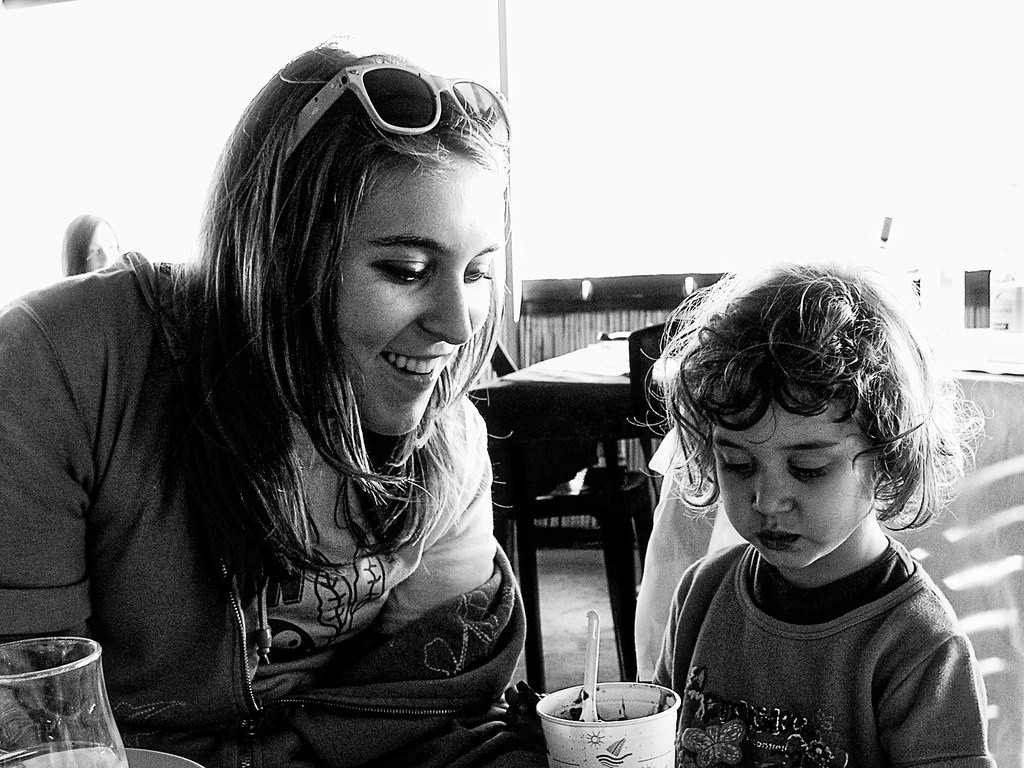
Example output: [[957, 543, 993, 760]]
[[536, 681, 682, 768], [0, 636, 130, 768]]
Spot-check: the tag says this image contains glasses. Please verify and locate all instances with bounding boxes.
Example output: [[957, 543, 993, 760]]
[[283, 64, 512, 162]]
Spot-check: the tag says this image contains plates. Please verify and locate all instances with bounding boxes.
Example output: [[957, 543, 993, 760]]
[[9, 746, 205, 768]]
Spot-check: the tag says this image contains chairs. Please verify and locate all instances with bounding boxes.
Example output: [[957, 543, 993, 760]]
[[469, 345, 641, 686], [628, 317, 681, 453]]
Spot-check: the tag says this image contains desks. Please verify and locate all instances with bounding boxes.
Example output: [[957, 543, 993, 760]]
[[481, 337, 650, 688], [630, 301, 1024, 768]]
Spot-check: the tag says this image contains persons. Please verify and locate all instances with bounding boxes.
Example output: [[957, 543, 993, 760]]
[[627, 256, 1001, 768], [60, 215, 121, 279], [1, 45, 548, 768]]
